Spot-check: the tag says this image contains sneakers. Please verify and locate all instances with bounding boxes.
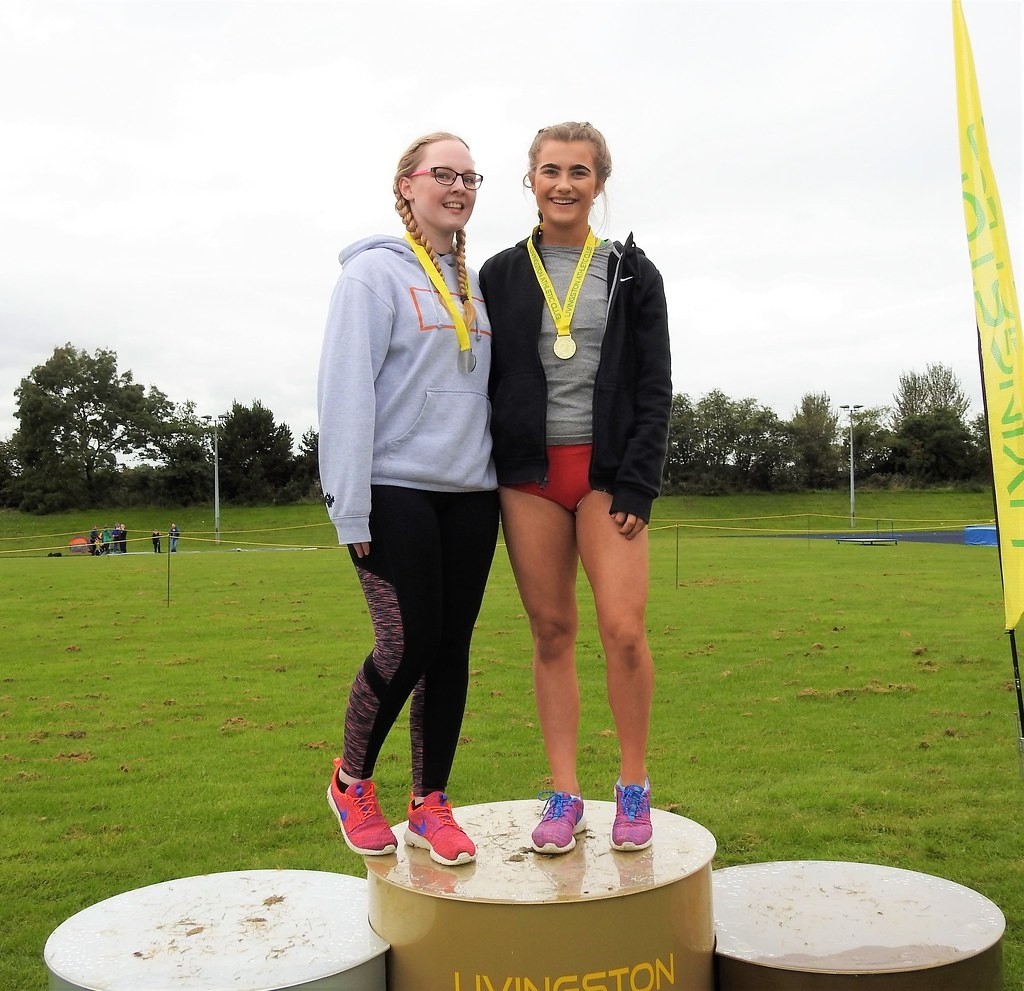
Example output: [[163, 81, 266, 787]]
[[530, 788, 588, 853], [325, 757, 398, 856], [402, 791, 478, 865], [610, 773, 654, 851]]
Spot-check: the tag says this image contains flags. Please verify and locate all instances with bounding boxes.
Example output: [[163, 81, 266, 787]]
[[952, 0, 1024, 632]]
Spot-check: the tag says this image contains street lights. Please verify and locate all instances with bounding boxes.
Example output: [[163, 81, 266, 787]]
[[840, 404, 864, 528], [201, 414, 230, 544]]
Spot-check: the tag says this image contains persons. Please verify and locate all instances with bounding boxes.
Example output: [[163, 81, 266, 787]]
[[168, 524, 181, 553], [152, 529, 161, 553], [90, 522, 127, 556], [477, 122, 672, 854], [315, 131, 500, 868]]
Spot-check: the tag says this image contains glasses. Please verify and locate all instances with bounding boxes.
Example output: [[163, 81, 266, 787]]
[[409, 166, 485, 191]]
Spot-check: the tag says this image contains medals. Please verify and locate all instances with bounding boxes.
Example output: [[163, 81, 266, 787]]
[[553, 332, 576, 360], [457, 348, 477, 374]]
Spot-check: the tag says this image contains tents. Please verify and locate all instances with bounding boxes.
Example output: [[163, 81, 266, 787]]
[[70, 536, 91, 554]]
